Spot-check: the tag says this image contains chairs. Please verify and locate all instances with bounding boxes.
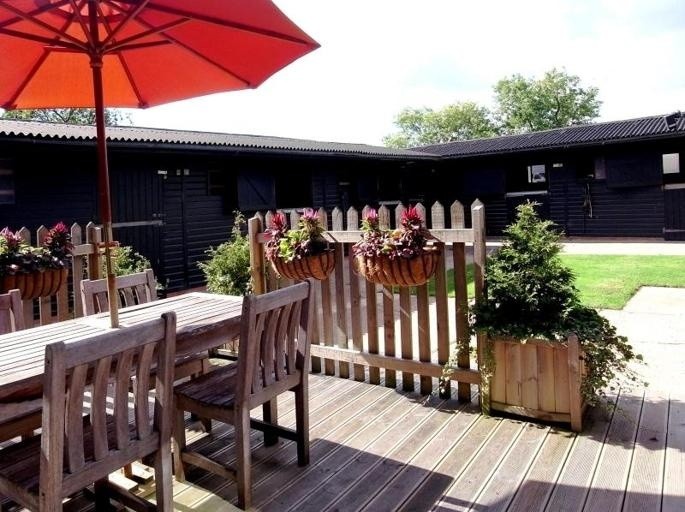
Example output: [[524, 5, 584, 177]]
[[80, 269, 213, 464], [0, 289, 71, 449], [1, 310, 176, 512], [172, 278, 314, 512]]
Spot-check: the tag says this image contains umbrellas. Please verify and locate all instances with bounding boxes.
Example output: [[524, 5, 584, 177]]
[[0, 0, 322, 327]]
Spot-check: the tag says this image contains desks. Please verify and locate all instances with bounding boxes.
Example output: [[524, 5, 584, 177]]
[[1, 292, 280, 448]]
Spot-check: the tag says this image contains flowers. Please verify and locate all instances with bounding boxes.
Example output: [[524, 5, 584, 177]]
[[263, 208, 326, 262], [351, 207, 444, 255], [0, 220, 75, 274]]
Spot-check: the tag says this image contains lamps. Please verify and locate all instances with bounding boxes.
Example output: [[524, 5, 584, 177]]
[[662, 153, 680, 175]]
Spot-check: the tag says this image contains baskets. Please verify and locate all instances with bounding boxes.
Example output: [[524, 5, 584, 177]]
[[1, 269, 66, 301]]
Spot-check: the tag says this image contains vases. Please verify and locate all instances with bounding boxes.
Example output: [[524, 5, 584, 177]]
[[0, 266, 69, 300]]
